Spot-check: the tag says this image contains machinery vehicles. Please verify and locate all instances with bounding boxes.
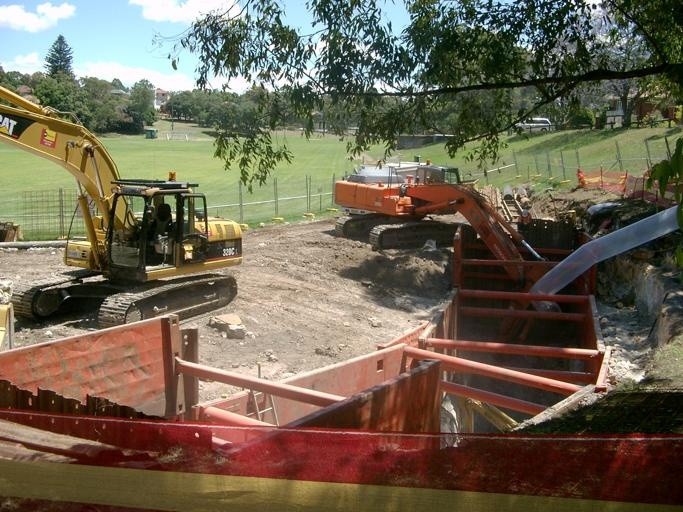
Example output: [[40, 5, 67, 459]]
[[0, 85, 244, 328], [332, 154, 584, 313]]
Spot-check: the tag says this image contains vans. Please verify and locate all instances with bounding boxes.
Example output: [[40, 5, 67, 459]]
[[514, 116, 552, 135]]
